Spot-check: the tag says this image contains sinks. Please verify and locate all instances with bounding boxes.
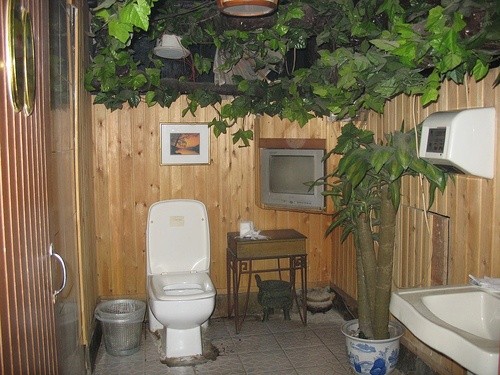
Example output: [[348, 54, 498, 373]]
[[389, 284, 500, 374]]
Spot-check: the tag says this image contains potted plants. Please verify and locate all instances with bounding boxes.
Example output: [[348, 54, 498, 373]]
[[303, 120, 454, 374]]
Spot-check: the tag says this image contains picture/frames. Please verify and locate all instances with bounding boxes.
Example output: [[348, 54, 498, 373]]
[[160, 123, 212, 165]]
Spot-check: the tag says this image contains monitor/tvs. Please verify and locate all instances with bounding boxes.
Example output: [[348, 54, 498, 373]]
[[259, 146, 325, 212]]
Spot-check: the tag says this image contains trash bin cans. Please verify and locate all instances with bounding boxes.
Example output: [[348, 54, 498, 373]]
[[95, 298, 147, 356]]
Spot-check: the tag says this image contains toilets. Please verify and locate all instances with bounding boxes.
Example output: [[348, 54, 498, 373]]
[[146, 198, 217, 356]]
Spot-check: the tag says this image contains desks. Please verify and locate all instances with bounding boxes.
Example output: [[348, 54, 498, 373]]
[[225, 229, 308, 334]]
